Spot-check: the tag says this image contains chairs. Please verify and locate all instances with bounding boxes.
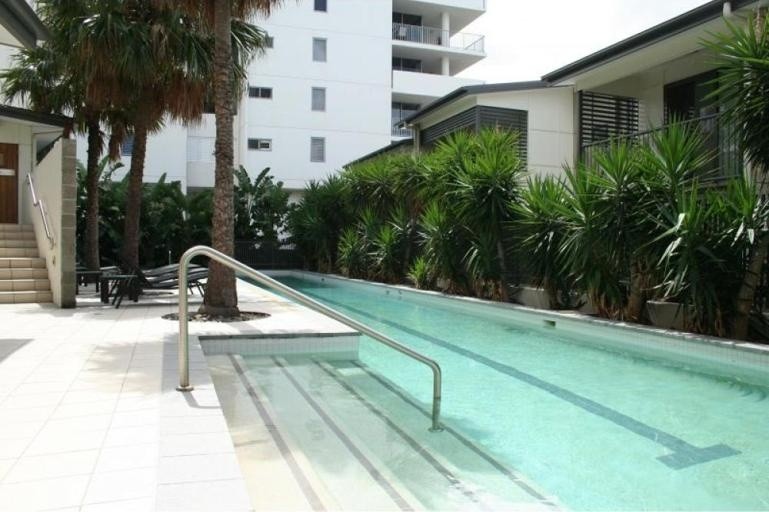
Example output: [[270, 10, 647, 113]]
[[76, 259, 209, 310]]
